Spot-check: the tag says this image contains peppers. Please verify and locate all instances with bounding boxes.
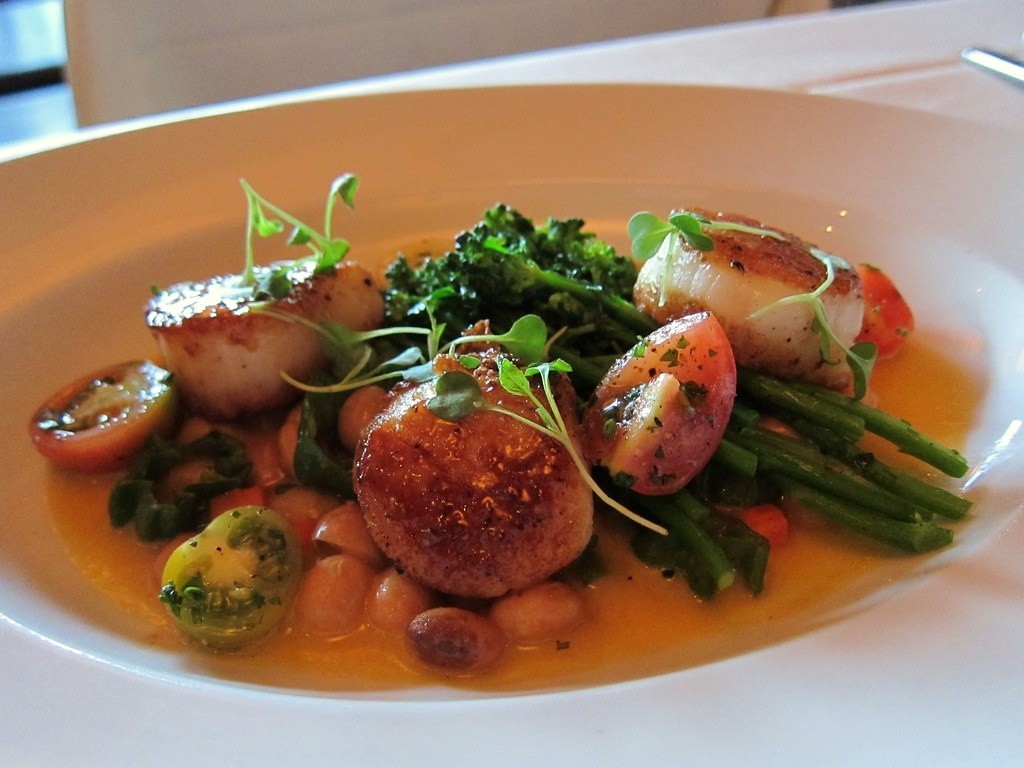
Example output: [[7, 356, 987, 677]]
[[108, 428, 253, 544]]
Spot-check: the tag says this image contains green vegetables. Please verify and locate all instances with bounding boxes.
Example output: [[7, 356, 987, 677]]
[[239, 172, 974, 598]]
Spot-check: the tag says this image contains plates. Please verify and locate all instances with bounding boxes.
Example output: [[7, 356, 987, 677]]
[[0, 85, 1024, 703]]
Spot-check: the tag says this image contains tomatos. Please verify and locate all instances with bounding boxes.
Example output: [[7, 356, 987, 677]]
[[154, 502, 300, 646], [27, 360, 183, 468]]
